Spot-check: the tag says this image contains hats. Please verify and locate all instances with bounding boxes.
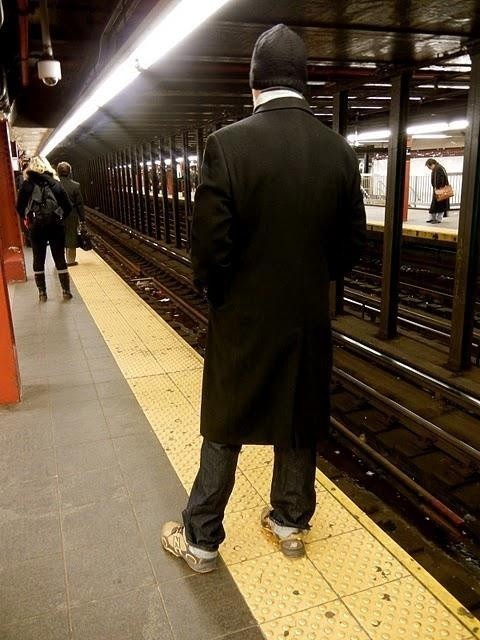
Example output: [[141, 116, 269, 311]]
[[248, 23, 310, 94]]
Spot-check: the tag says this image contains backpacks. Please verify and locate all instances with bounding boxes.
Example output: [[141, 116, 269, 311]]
[[23, 178, 66, 234]]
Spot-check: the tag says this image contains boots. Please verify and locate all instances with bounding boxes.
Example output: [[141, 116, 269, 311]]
[[34, 271, 47, 302], [57, 269, 73, 301]]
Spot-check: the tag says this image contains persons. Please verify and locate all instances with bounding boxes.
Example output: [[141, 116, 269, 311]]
[[424, 158, 449, 223], [159, 23, 368, 575], [17, 157, 88, 302]]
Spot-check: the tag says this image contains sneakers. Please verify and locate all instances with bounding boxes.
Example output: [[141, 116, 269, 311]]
[[426, 219, 441, 224], [261, 506, 306, 559], [160, 520, 219, 574], [66, 262, 79, 266]]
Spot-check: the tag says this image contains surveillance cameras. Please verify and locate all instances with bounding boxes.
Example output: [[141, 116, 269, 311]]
[[37, 60, 62, 87]]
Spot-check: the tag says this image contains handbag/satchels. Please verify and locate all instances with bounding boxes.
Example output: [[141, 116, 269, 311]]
[[78, 230, 93, 251], [434, 185, 454, 202]]
